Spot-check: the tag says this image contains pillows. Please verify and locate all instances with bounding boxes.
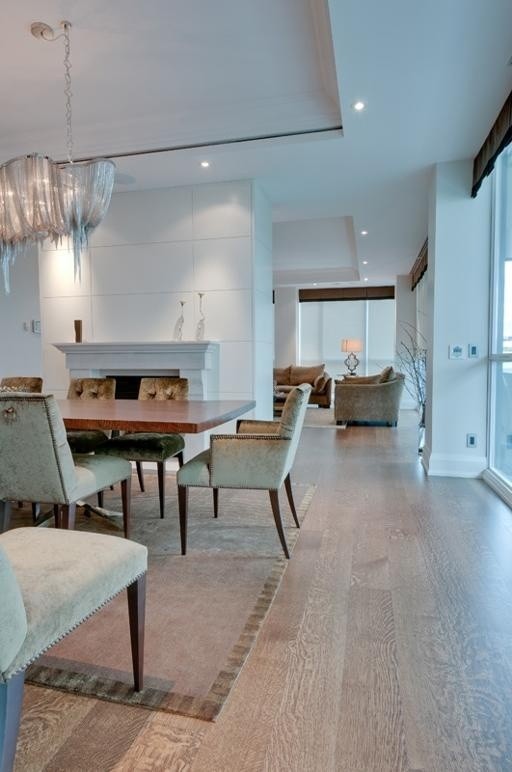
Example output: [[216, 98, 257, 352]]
[[376, 366, 396, 384], [274, 363, 326, 385]]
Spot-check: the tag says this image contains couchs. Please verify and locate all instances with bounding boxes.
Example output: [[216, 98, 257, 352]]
[[274, 371, 332, 407]]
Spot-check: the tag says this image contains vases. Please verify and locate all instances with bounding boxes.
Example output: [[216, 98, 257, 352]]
[[418, 405, 426, 454]]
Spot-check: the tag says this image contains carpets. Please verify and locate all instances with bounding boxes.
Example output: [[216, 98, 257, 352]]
[[274, 407, 348, 429], [0, 467, 319, 723]]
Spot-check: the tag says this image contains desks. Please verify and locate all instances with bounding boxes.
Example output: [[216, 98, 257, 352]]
[[32, 399, 256, 528]]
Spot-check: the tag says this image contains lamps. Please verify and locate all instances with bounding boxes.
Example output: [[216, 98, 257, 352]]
[[341, 338, 363, 376], [0, 21, 116, 297]]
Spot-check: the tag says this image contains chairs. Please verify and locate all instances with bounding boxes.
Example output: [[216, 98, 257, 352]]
[[0, 526, 149, 772], [177, 381, 313, 559], [94, 378, 190, 519], [335, 372, 405, 427], [0, 377, 132, 540]]
